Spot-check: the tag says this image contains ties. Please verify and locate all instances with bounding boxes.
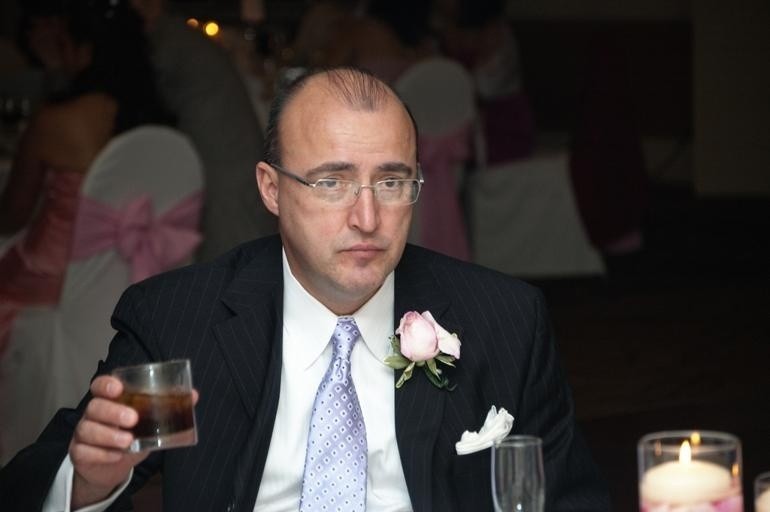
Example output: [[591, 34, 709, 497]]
[[299, 318, 367, 511]]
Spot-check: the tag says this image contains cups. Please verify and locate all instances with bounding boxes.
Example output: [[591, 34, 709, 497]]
[[492, 434, 547, 512], [637, 429, 745, 512], [112, 358, 198, 453]]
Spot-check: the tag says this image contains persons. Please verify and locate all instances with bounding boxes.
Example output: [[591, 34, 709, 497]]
[[1, 65, 616, 511], [0, 1, 536, 344]]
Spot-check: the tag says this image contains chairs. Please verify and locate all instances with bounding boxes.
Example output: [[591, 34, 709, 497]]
[[6, 124, 204, 454], [396, 55, 475, 263]]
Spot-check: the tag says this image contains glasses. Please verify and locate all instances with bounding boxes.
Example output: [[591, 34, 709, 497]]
[[267, 161, 426, 206]]
